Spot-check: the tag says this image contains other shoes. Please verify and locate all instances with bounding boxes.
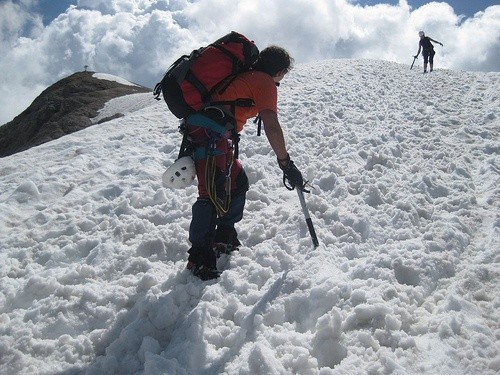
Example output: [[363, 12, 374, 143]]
[[187, 258, 219, 280], [214, 227, 243, 251]]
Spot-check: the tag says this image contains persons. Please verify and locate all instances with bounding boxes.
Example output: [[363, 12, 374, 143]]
[[414, 31, 443, 73], [178, 46, 303, 281]]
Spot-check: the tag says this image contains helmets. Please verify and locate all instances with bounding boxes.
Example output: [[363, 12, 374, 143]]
[[419, 31, 425, 39], [161, 155, 197, 191]]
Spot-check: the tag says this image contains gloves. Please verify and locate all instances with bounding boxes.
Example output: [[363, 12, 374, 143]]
[[281, 160, 311, 193]]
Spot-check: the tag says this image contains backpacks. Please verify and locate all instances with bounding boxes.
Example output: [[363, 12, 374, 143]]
[[159, 30, 259, 119]]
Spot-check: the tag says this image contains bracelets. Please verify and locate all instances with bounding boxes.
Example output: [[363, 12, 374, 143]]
[[277, 154, 290, 169]]
